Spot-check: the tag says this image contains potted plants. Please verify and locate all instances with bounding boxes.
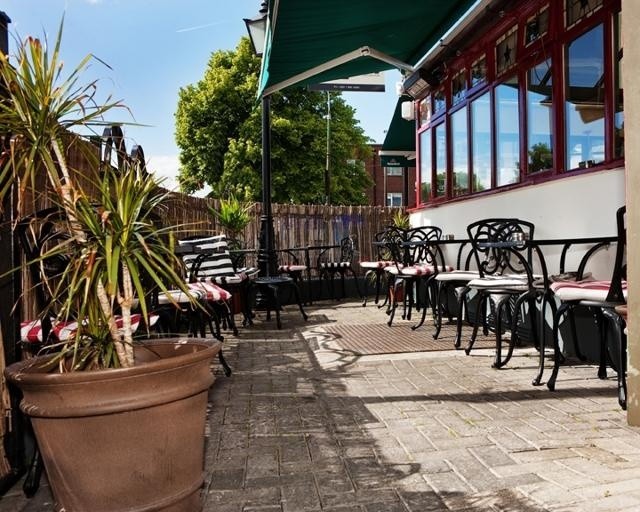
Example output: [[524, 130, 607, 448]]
[[0, 12, 220, 511]]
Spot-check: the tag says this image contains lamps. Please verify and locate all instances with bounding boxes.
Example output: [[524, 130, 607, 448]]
[[401, 101, 414, 121]]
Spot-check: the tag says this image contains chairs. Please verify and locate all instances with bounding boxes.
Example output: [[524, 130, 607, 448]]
[[242, 247, 308, 328], [12, 205, 238, 494], [317, 236, 362, 303], [361, 208, 625, 407]]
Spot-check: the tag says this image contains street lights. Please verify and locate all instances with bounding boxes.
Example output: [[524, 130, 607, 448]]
[[244, 0, 291, 284]]
